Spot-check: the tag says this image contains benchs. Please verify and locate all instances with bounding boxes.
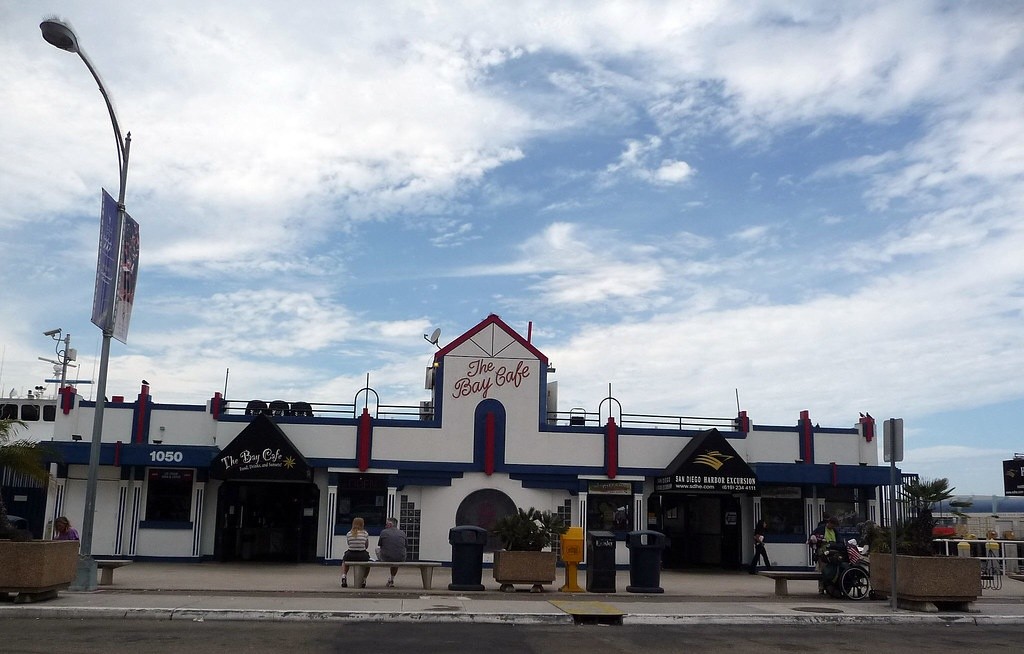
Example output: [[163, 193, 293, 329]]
[[94, 559, 133, 585], [758, 570, 825, 596], [345, 561, 442, 590]]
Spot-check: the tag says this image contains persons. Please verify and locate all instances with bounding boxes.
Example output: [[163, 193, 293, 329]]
[[808, 512, 865, 597], [341, 518, 371, 588], [54, 517, 79, 541], [376, 518, 408, 587], [7, 520, 33, 542], [748, 521, 772, 575]]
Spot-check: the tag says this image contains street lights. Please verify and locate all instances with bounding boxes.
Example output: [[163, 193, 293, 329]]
[[39, 13, 132, 593]]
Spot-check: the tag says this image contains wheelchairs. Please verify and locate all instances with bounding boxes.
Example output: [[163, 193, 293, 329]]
[[810, 539, 870, 601]]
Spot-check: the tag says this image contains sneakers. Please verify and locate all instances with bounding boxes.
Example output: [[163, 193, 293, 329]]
[[361, 580, 366, 588], [386, 580, 394, 587], [341, 577, 348, 588]]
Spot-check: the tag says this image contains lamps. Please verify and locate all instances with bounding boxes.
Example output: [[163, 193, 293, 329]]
[[795, 459, 804, 464], [859, 462, 868, 466], [72, 435, 82, 442], [152, 440, 163, 445]]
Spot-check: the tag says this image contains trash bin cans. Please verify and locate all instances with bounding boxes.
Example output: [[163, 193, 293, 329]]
[[557, 527, 589, 594], [625, 530, 667, 594], [586, 530, 617, 594], [447, 525, 488, 591]]
